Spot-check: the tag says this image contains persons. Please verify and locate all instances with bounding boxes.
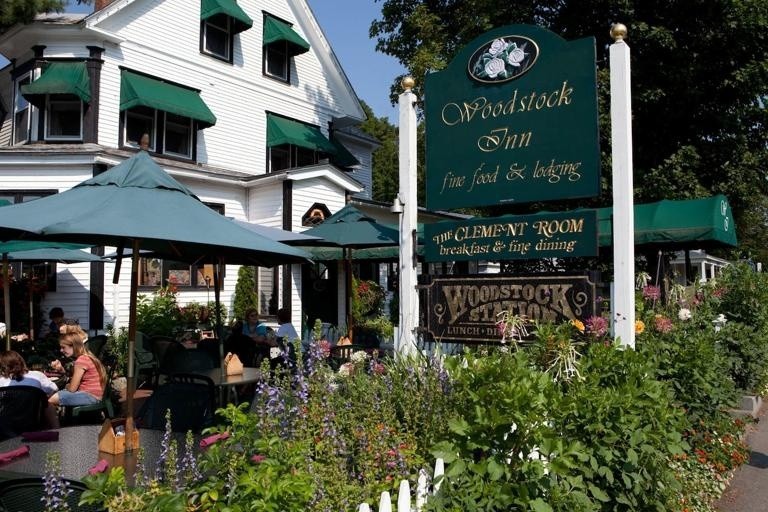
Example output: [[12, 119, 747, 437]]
[[1, 307, 109, 426], [163, 309, 305, 370]]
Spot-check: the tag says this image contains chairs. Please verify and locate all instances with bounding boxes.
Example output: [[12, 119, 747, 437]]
[[0, 326, 366, 512]]
